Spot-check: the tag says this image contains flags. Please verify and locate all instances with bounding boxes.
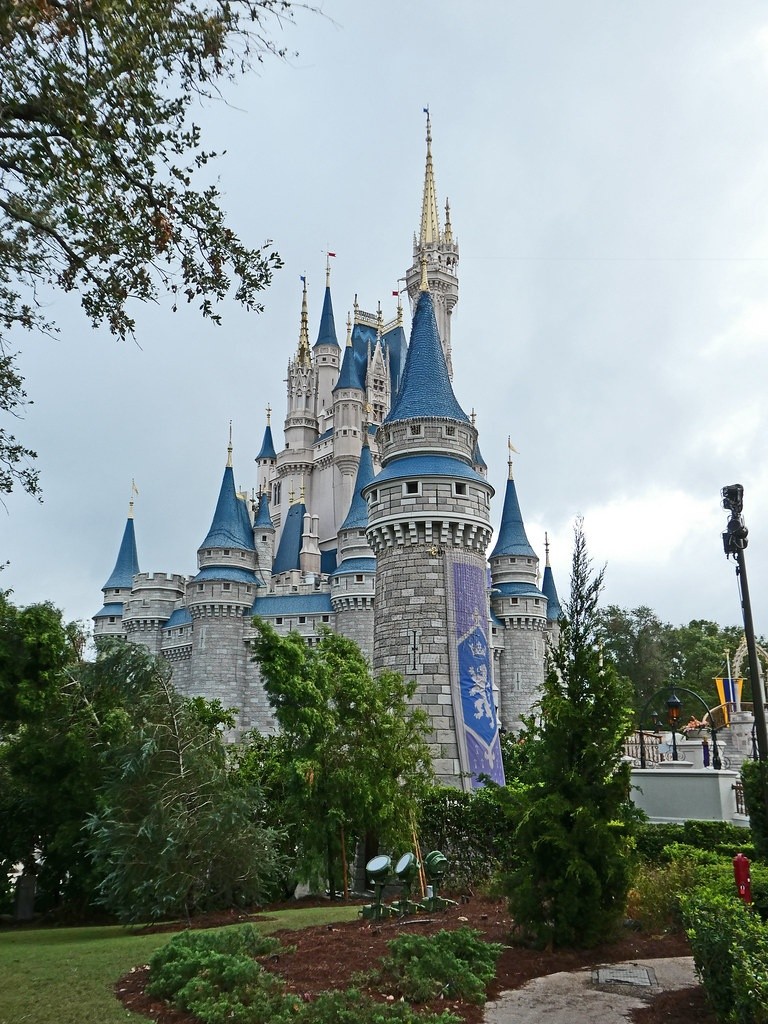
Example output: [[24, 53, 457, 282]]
[[329, 253, 336, 257], [301, 276, 305, 281], [393, 291, 398, 296], [508, 438, 520, 454], [132, 483, 138, 494]]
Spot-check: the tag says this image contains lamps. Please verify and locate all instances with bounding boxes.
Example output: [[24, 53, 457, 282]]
[[358, 851, 455, 919]]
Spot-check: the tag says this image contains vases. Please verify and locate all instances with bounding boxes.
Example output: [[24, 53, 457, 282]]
[[687, 729, 708, 741]]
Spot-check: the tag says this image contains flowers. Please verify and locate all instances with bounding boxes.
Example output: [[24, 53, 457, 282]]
[[677, 716, 710, 733]]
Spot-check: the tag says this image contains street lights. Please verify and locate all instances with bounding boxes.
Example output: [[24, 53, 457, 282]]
[[663, 690, 683, 762], [648, 711, 662, 734]]
